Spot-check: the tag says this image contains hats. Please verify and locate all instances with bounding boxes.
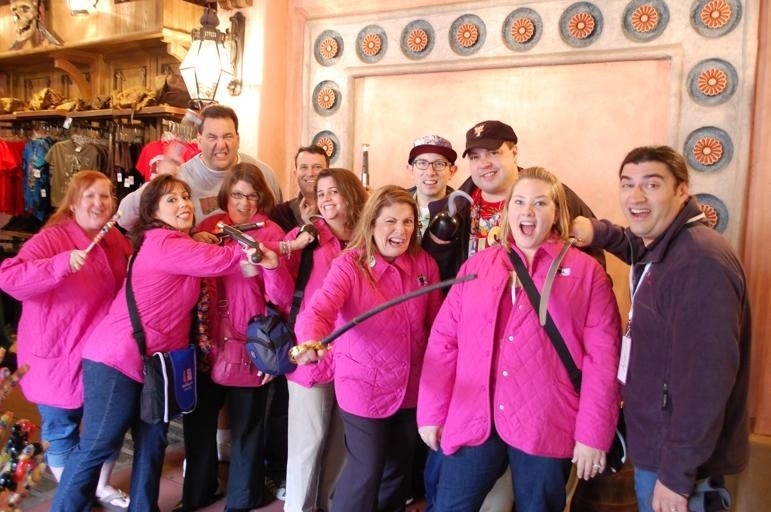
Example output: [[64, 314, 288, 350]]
[[409, 132, 458, 163], [461, 119, 517, 158]]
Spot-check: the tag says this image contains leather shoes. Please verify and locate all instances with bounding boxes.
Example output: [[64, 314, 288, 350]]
[[224, 498, 258, 512], [171, 493, 208, 511]]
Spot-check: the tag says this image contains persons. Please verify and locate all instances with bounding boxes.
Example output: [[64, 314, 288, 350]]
[[567, 144, 753, 512], [2, 100, 624, 512]]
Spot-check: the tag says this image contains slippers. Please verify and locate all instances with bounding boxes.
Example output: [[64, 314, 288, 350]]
[[99, 484, 131, 509]]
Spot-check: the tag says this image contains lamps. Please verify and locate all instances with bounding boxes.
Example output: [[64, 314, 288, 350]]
[[179, 6, 246, 110]]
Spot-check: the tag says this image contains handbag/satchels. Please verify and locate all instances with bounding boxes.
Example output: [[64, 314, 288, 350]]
[[243, 312, 298, 378], [139, 342, 199, 424], [208, 319, 264, 387], [601, 411, 626, 478]]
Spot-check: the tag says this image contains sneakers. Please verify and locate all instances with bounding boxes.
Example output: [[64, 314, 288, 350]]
[[265, 470, 286, 500]]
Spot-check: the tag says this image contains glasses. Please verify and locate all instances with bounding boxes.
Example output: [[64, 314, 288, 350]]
[[229, 192, 261, 201], [413, 158, 453, 172]]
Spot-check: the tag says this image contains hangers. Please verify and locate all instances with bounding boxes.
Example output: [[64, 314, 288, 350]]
[[1, 122, 200, 147]]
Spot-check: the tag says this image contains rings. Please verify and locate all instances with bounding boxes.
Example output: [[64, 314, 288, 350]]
[[670, 508, 677, 511]]
